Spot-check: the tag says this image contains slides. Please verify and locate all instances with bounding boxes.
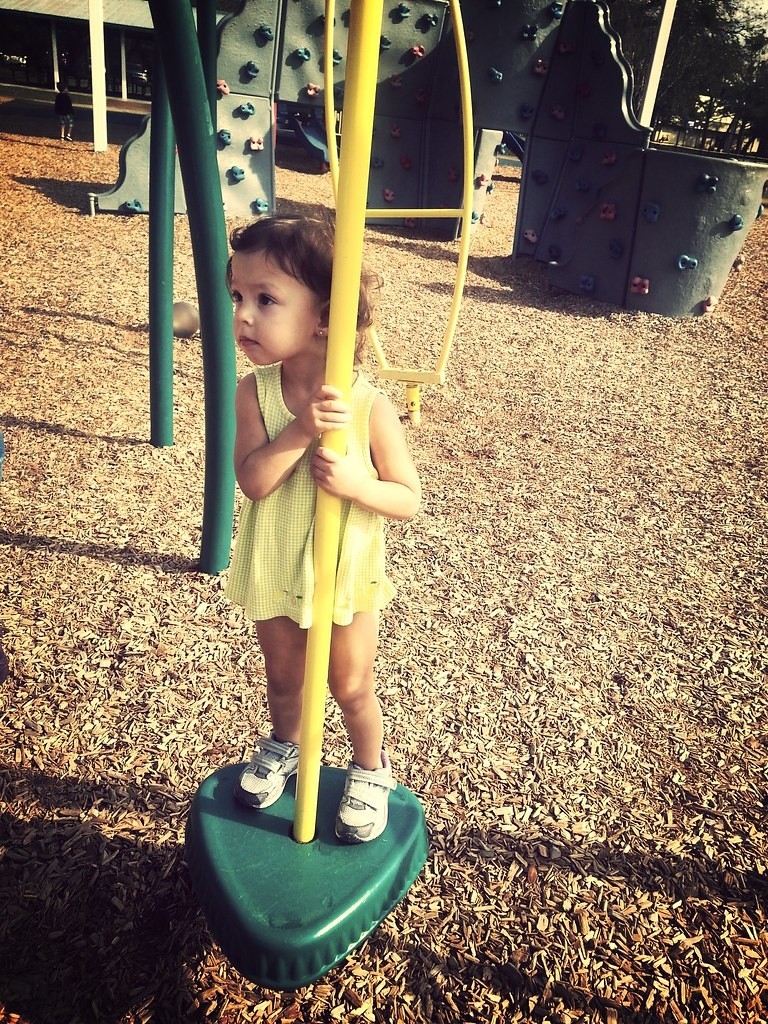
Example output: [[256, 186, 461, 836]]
[[284, 102, 341, 163]]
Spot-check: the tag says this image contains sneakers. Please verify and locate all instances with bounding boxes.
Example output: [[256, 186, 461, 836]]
[[335, 749, 398, 844], [234, 731, 299, 811]]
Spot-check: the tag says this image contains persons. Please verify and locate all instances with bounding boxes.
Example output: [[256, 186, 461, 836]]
[[227, 216, 422, 842]]
